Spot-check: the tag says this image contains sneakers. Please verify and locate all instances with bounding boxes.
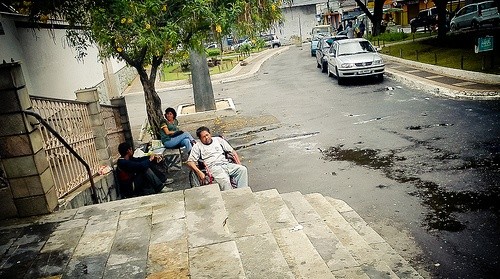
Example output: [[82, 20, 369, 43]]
[[163, 179, 174, 185], [161, 186, 173, 193]]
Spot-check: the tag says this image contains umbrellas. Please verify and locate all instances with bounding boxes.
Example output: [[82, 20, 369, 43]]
[[342, 15, 357, 30], [357, 5, 404, 19]]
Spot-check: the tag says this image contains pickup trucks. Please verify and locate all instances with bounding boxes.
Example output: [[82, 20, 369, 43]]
[[410, 7, 454, 31]]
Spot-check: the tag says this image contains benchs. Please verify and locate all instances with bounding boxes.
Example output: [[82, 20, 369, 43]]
[[163, 148, 182, 173]]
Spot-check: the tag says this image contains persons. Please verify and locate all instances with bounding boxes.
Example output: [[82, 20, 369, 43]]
[[159, 107, 196, 156], [115, 142, 174, 199], [187, 125, 248, 191], [336, 20, 366, 39]]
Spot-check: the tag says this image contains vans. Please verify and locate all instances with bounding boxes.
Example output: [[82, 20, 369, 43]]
[[309, 25, 334, 57], [450, 1, 500, 31]]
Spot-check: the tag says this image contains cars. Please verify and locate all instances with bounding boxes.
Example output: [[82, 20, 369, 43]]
[[326, 38, 386, 85], [315, 35, 349, 73], [206, 33, 281, 51]]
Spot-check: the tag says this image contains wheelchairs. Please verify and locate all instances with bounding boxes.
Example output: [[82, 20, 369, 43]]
[[188, 140, 239, 188]]
